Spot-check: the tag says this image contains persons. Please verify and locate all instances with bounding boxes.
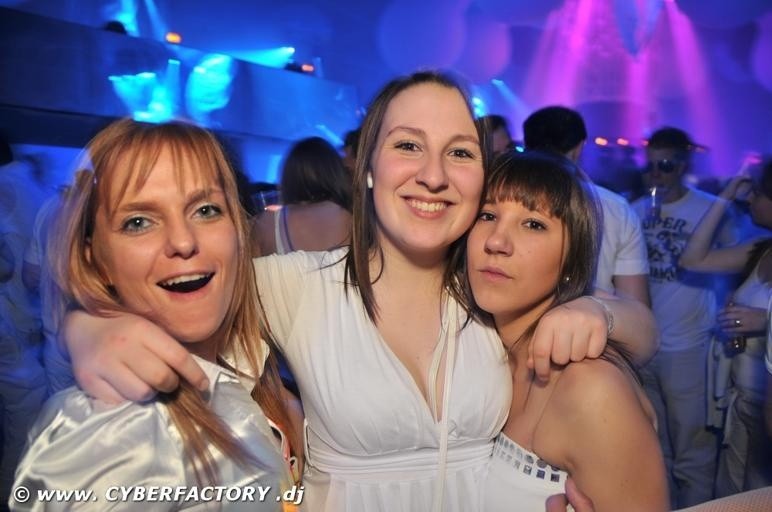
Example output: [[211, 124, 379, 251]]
[[6, 67, 772, 512]]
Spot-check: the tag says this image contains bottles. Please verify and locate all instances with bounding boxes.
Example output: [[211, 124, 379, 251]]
[[722, 300, 746, 358]]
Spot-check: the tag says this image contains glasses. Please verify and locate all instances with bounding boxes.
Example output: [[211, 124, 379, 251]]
[[637, 158, 678, 174]]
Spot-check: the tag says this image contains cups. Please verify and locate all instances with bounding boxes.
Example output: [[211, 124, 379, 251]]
[[644, 182, 664, 221]]
